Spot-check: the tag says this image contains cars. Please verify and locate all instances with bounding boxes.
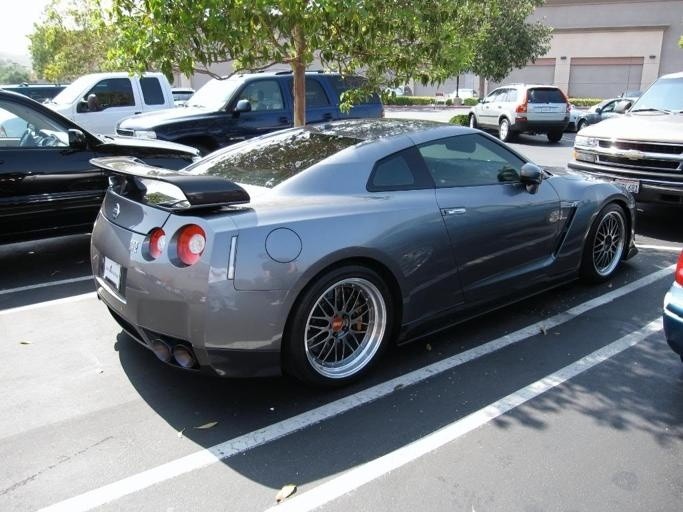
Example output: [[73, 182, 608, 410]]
[[385, 85, 413, 97], [566, 71, 682, 224], [0, 70, 198, 140], [0, 88, 203, 245]]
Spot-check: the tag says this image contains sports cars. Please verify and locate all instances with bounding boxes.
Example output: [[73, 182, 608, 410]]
[[87, 114, 640, 392]]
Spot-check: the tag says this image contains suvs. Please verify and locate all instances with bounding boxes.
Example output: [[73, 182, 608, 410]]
[[114, 68, 384, 158], [467, 82, 571, 143]]
